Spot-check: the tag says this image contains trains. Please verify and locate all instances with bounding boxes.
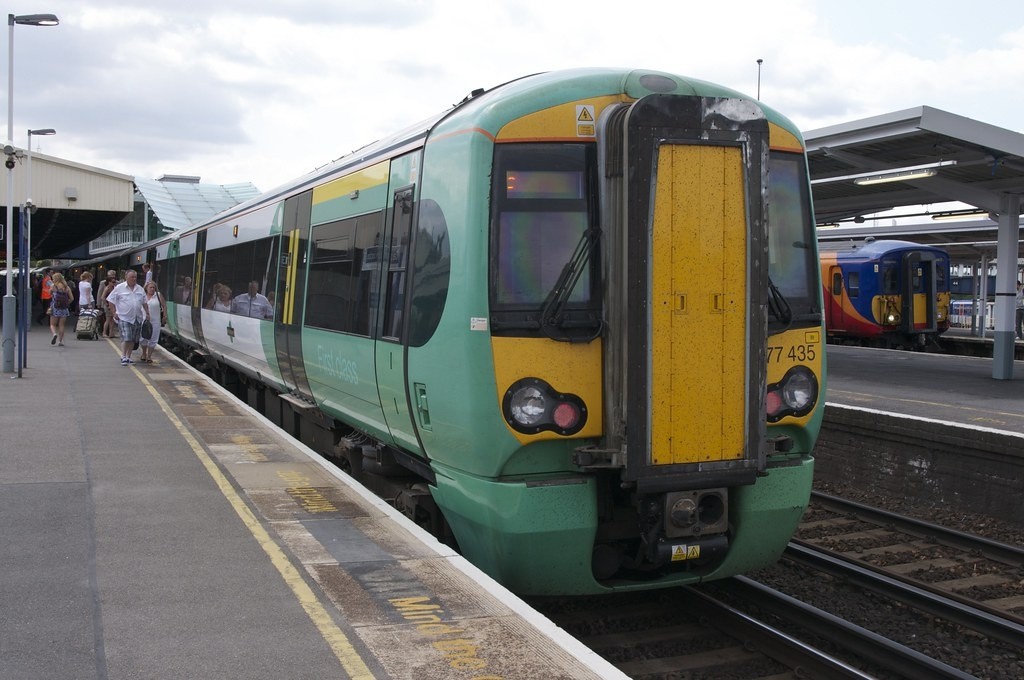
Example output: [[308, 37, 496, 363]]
[[817, 237, 952, 351], [0, 67, 828, 601]]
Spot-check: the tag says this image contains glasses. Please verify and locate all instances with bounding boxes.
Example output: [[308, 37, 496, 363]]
[[112, 281, 116, 284]]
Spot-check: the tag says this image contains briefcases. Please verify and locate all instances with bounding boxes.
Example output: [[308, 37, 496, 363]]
[[76, 306, 99, 340]]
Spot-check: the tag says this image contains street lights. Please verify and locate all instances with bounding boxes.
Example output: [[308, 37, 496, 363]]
[[2, 12, 60, 373], [26, 128, 56, 332]]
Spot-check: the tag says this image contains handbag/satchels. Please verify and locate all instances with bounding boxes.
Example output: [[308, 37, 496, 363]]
[[160, 310, 166, 328], [141, 319, 153, 340]]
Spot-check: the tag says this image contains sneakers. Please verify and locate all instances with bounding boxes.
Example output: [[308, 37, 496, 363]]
[[121, 356, 127, 366], [126, 357, 136, 365]]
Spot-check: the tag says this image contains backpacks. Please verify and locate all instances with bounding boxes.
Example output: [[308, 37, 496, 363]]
[[53, 285, 69, 308]]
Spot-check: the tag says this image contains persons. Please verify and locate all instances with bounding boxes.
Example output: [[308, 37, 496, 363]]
[[36, 263, 167, 366], [176, 276, 192, 305], [206, 281, 283, 322], [1014, 280, 1024, 341]]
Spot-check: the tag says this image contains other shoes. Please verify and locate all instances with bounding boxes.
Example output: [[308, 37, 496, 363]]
[[141, 356, 146, 361], [147, 359, 153, 363], [59, 341, 64, 346], [51, 334, 58, 344]]
